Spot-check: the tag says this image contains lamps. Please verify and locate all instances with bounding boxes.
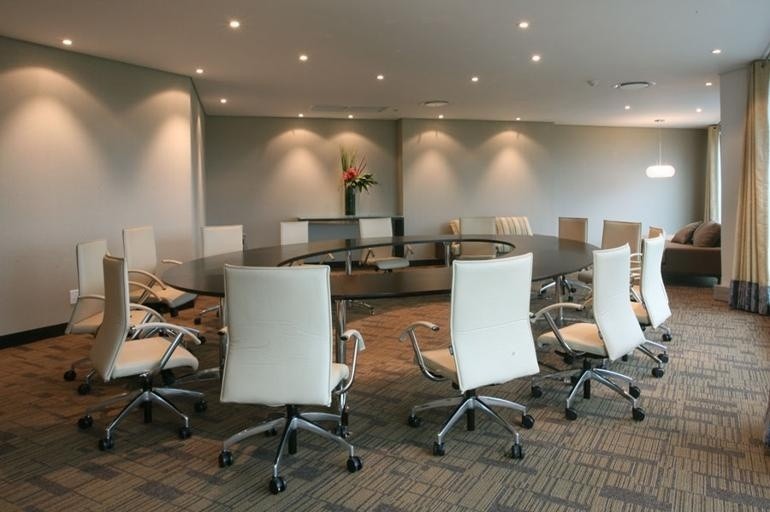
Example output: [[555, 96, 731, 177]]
[[646, 120, 675, 178]]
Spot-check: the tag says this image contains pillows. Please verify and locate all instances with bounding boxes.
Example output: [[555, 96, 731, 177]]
[[691, 221, 721, 248], [670, 220, 703, 244]]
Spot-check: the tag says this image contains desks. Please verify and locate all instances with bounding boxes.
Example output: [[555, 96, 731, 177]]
[[297, 216, 404, 265]]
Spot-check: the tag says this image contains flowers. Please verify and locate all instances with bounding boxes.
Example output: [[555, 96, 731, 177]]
[[339, 143, 378, 194]]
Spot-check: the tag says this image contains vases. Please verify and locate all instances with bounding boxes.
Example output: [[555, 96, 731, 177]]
[[345, 188, 359, 215]]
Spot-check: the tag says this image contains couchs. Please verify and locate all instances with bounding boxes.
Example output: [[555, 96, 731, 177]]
[[658, 238, 721, 286]]
